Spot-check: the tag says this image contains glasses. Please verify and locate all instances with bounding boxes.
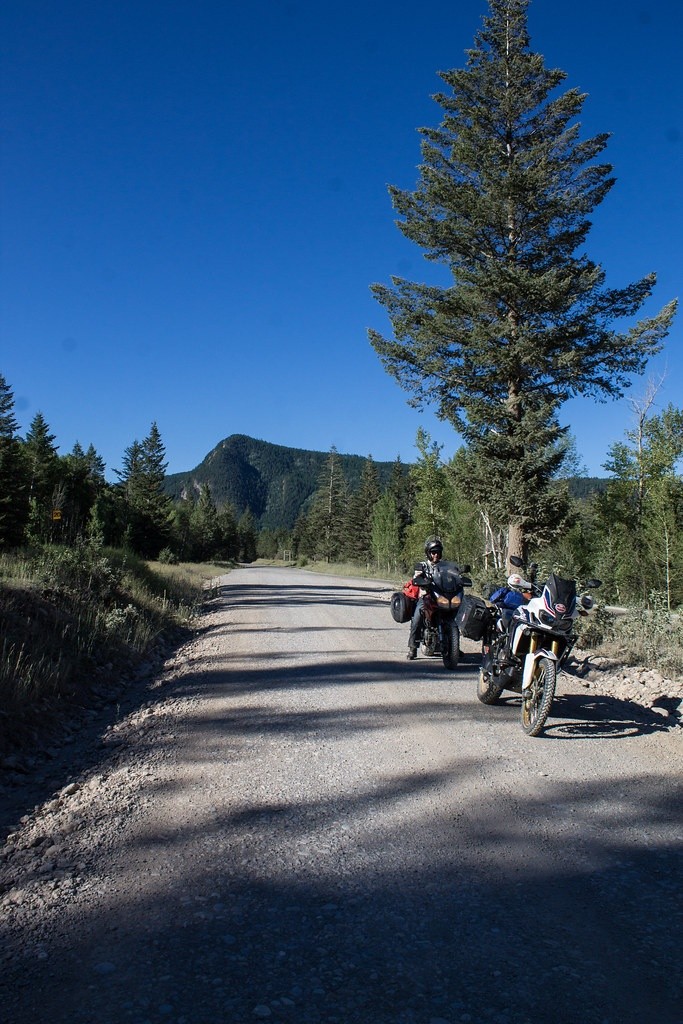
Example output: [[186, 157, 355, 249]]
[[430, 551, 440, 555]]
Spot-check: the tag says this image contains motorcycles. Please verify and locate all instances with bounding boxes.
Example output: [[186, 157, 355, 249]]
[[412, 563, 471, 666], [477, 555, 601, 735]]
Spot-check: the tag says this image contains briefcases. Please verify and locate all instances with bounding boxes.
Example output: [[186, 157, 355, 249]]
[[453, 594, 490, 642]]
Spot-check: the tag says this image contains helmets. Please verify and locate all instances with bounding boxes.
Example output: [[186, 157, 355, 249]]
[[425, 540, 443, 561]]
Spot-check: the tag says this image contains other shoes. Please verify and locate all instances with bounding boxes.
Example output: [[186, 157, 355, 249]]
[[406, 649, 417, 660]]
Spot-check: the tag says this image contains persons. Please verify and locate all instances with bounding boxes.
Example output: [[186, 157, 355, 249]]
[[406, 539, 465, 659]]
